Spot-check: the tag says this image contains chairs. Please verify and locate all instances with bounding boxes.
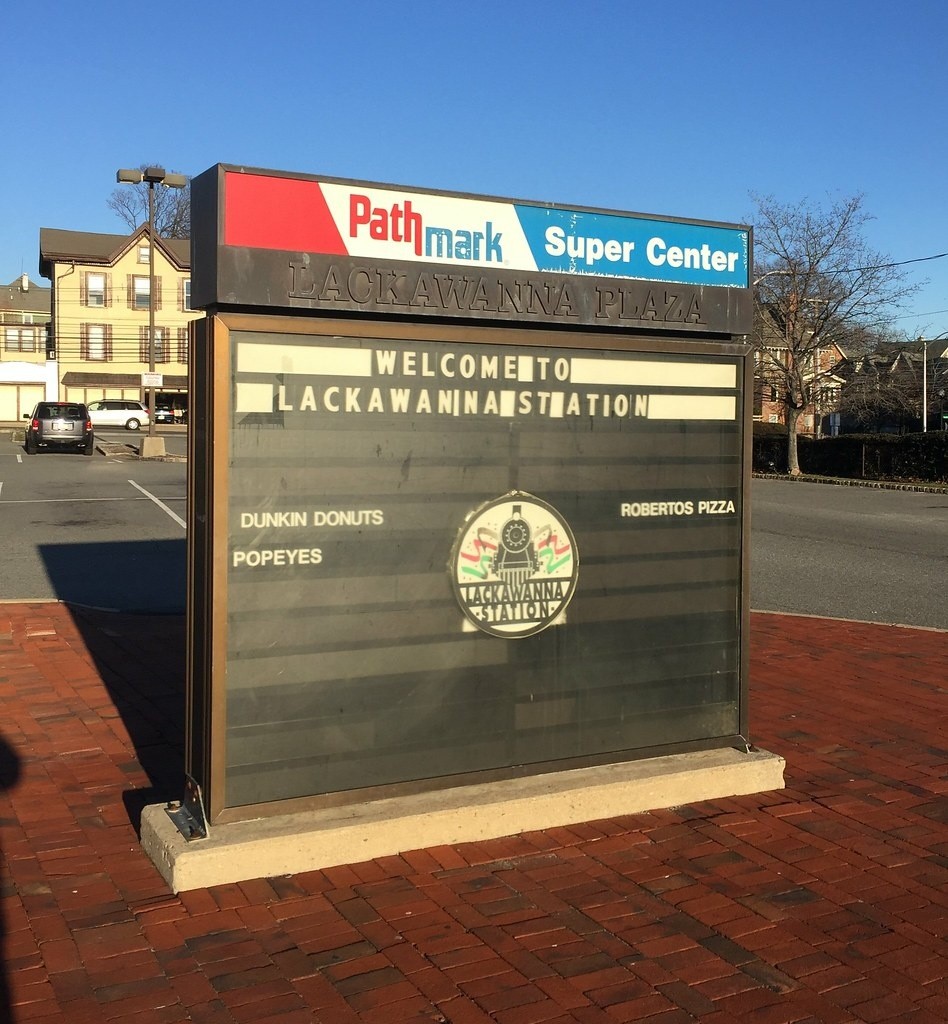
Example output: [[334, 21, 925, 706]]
[[67, 409, 79, 419], [38, 408, 50, 418]]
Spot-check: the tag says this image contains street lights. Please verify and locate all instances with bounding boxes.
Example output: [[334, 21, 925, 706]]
[[116, 166, 188, 438]]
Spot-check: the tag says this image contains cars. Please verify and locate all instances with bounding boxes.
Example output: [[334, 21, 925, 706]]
[[155, 402, 175, 424], [24, 401, 94, 456]]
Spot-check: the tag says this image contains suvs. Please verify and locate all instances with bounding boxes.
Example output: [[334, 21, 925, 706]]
[[86, 398, 150, 430]]
